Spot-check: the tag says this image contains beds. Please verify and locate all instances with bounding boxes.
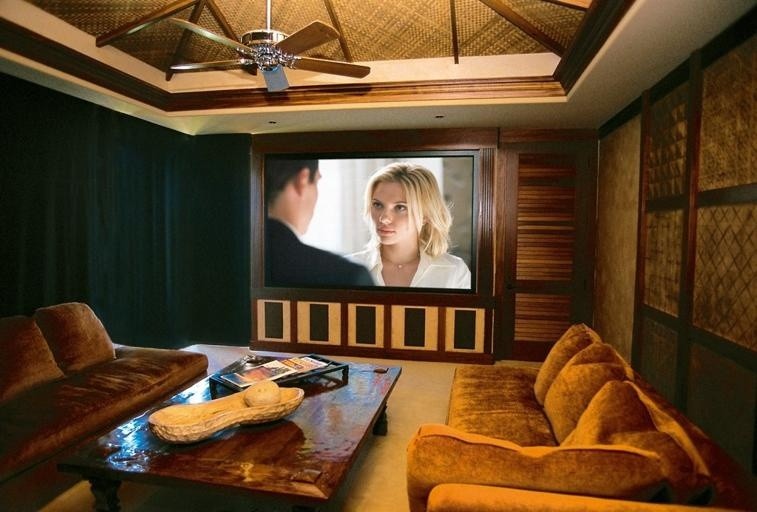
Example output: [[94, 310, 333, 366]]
[[0, 343, 209, 512]]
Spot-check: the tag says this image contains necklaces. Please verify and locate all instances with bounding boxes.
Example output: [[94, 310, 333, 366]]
[[381, 253, 420, 269]]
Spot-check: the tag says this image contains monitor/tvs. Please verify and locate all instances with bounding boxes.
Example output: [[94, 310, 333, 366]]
[[264, 150, 479, 293]]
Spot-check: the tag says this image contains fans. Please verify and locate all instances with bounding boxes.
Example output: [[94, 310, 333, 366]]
[[169, 0, 370, 95]]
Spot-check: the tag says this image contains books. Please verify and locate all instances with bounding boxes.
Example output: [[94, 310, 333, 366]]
[[220, 355, 328, 388]]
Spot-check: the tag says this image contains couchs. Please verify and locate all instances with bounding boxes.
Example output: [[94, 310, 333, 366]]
[[427, 360, 757, 512]]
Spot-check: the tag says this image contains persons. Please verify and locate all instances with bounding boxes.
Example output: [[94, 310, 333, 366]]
[[347, 162, 472, 289], [265, 158, 375, 290]]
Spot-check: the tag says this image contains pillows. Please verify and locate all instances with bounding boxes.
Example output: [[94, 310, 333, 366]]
[[533, 320, 604, 406], [558, 379, 716, 505], [33, 301, 117, 378], [404, 421, 667, 511], [542, 341, 635, 445], [0, 315, 65, 408]]
